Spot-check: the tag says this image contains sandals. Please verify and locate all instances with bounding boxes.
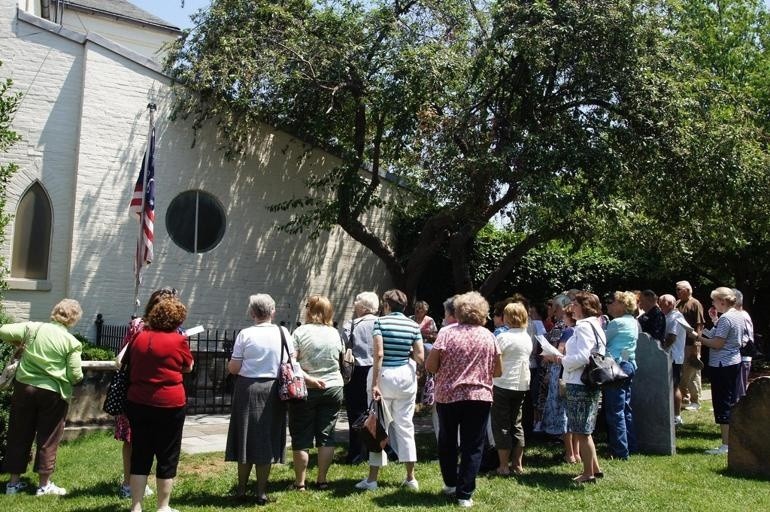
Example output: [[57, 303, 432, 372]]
[[233, 494, 247, 503], [256, 494, 267, 507]]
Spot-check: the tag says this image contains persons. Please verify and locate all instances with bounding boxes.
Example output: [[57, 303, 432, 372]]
[[114, 287, 186, 498], [125, 299, 194, 512], [0, 298, 83, 497]]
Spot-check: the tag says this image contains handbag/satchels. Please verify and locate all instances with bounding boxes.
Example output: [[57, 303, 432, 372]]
[[102, 329, 143, 417], [340, 318, 356, 385], [575, 320, 631, 390], [277, 325, 308, 403], [350, 396, 390, 453], [0, 321, 37, 388], [739, 340, 757, 357]]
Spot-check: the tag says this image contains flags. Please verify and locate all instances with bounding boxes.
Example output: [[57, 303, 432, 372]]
[[128, 124, 157, 284]]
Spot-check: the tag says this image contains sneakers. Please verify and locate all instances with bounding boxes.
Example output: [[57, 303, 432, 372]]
[[121, 481, 155, 498], [442, 484, 457, 495], [35, 481, 66, 497], [673, 402, 729, 455], [156, 506, 180, 512], [6, 481, 28, 495], [458, 497, 473, 508], [513, 467, 523, 473], [496, 467, 511, 474], [403, 479, 419, 490], [559, 450, 630, 484], [316, 481, 329, 490], [354, 478, 377, 491], [285, 482, 306, 491]]
